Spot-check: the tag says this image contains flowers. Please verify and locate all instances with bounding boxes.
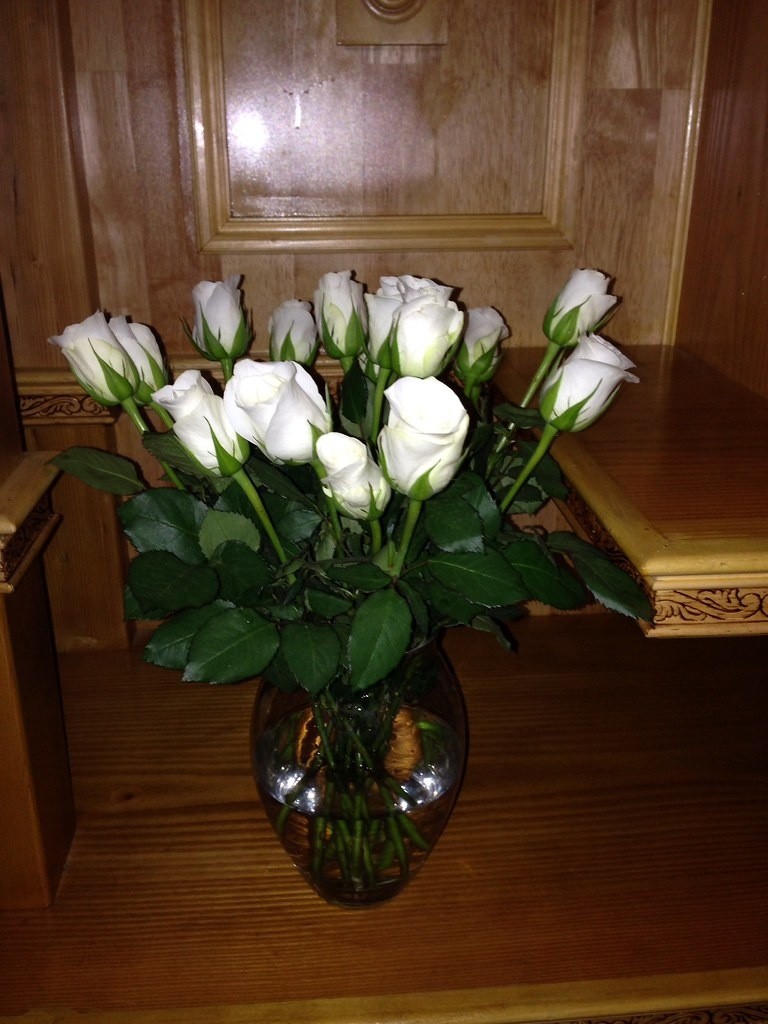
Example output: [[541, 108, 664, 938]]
[[46, 268, 640, 894]]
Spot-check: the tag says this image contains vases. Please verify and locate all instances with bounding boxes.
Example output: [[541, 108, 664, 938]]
[[251, 633, 469, 909]]
